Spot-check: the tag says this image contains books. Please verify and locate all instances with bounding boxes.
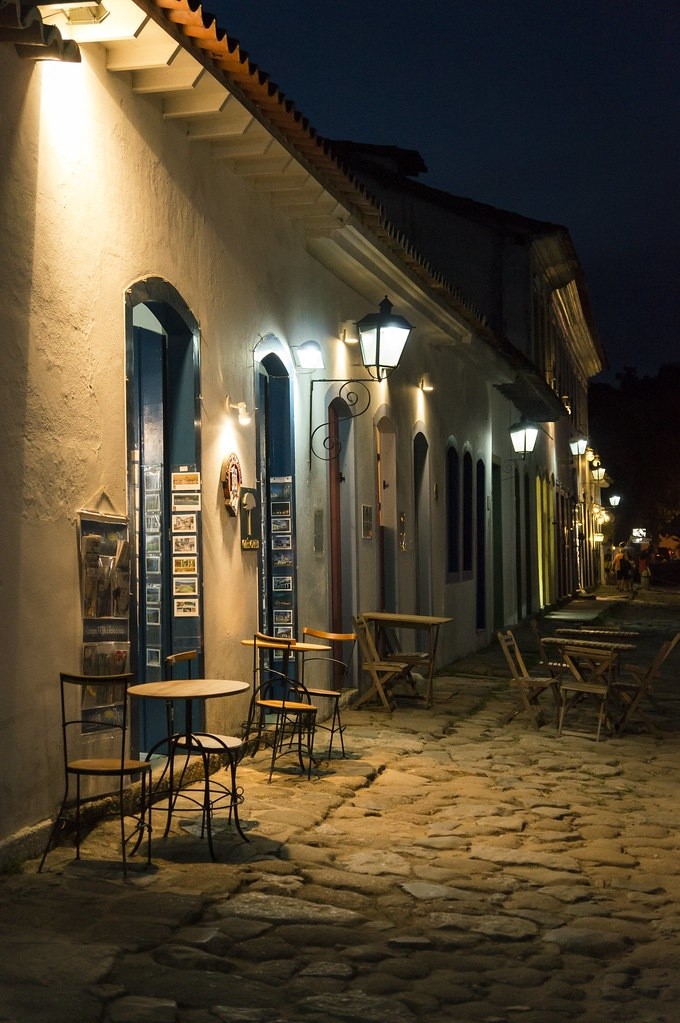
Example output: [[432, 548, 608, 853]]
[[81, 534, 131, 707]]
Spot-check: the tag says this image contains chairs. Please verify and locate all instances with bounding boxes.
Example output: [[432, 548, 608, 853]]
[[557, 643, 612, 743], [497, 630, 557, 729], [236, 632, 317, 782], [163, 649, 243, 838], [36, 672, 152, 877], [352, 613, 408, 712], [610, 633, 680, 737], [378, 609, 431, 704], [531, 618, 570, 705], [288, 627, 357, 756]]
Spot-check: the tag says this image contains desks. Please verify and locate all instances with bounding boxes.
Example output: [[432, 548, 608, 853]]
[[361, 611, 453, 708], [240, 639, 332, 770], [126, 679, 250, 859], [540, 637, 637, 730], [555, 627, 640, 643]]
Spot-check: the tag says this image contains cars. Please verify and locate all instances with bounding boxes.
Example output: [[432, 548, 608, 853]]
[[643, 546, 675, 560]]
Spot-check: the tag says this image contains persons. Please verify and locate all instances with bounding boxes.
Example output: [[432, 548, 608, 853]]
[[611, 549, 651, 592]]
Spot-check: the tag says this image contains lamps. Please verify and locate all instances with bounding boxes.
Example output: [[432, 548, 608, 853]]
[[605, 495, 620, 509], [590, 462, 605, 484], [587, 448, 595, 461], [419, 370, 434, 391], [501, 415, 539, 481], [309, 294, 416, 472], [569, 423, 587, 456], [294, 341, 325, 371], [339, 319, 359, 343], [593, 455, 600, 467]]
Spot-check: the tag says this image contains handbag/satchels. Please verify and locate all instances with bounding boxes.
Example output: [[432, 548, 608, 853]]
[[628, 570, 633, 576]]
